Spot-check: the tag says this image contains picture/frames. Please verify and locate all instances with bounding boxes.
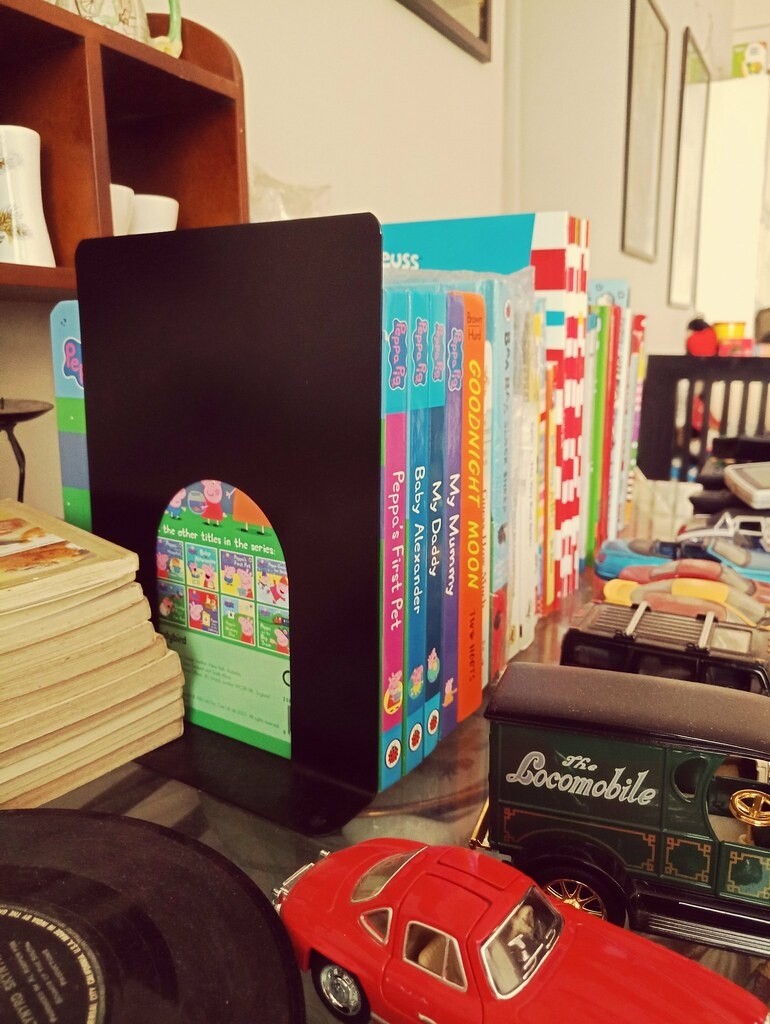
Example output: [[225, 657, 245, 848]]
[[621, 0, 670, 262], [395, 0, 493, 64], [667, 28, 713, 311]]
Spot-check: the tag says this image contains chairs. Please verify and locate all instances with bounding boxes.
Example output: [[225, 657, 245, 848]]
[[416, 933, 520, 995], [636, 354, 770, 482]]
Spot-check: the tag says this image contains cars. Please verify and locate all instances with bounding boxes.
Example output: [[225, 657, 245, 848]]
[[271, 525, 768, 1024]]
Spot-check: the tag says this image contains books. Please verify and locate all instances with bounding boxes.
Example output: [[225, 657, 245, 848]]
[[1, 209, 649, 810]]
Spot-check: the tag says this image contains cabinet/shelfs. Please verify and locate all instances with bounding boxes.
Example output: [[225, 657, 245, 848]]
[[0, 0, 248, 304]]
